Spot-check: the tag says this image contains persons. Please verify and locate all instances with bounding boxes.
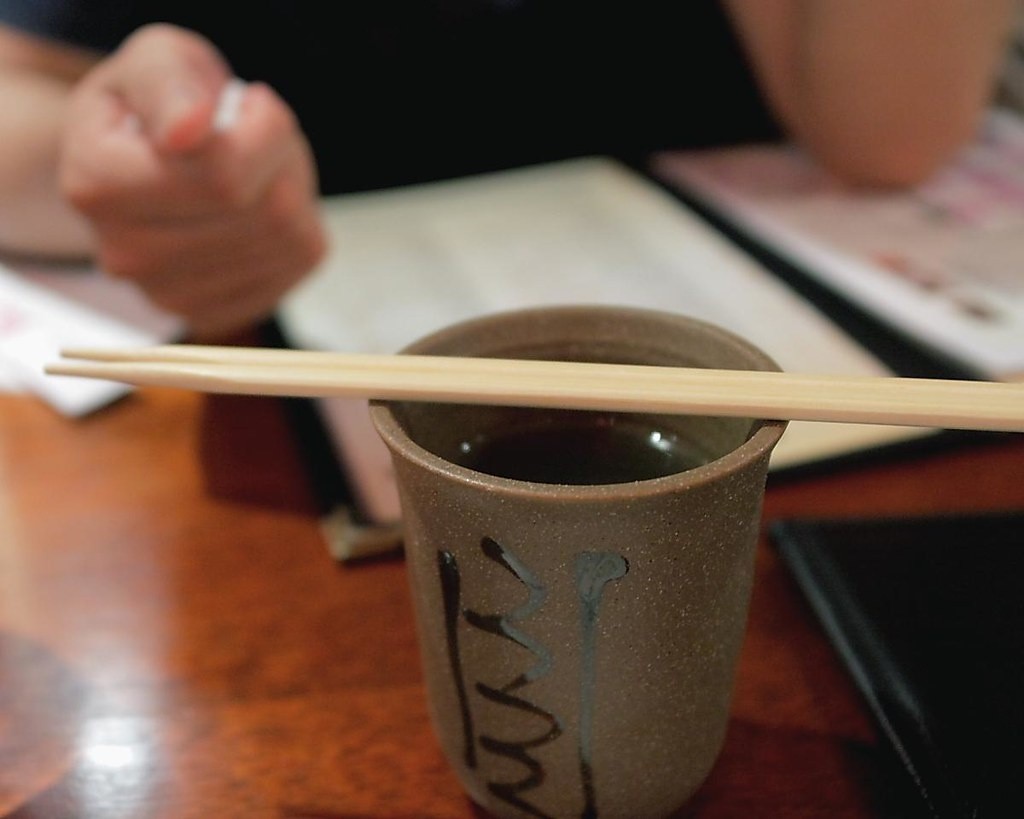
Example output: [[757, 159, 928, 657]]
[[0, 0, 1013, 343]]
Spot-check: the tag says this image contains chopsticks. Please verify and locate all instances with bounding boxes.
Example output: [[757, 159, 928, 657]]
[[44, 343, 1024, 433]]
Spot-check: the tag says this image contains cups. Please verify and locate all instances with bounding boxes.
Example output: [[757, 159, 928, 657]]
[[368, 304, 790, 819]]
